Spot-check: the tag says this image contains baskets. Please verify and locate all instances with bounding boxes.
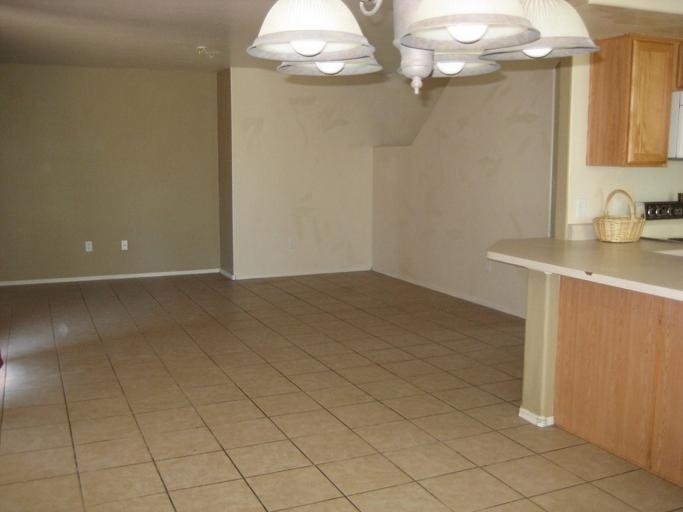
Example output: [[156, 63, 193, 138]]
[[592, 189, 645, 243]]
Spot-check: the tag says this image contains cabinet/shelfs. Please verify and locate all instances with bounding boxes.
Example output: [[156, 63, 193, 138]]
[[586, 33, 679, 168], [556, 274, 683, 490]]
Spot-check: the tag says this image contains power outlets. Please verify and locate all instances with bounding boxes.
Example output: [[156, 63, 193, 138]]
[[84, 241, 93, 253], [121, 240, 128, 251]]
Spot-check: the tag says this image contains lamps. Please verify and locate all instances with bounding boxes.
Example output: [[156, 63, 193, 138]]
[[390, 1, 594, 95], [246, 1, 383, 83]]
